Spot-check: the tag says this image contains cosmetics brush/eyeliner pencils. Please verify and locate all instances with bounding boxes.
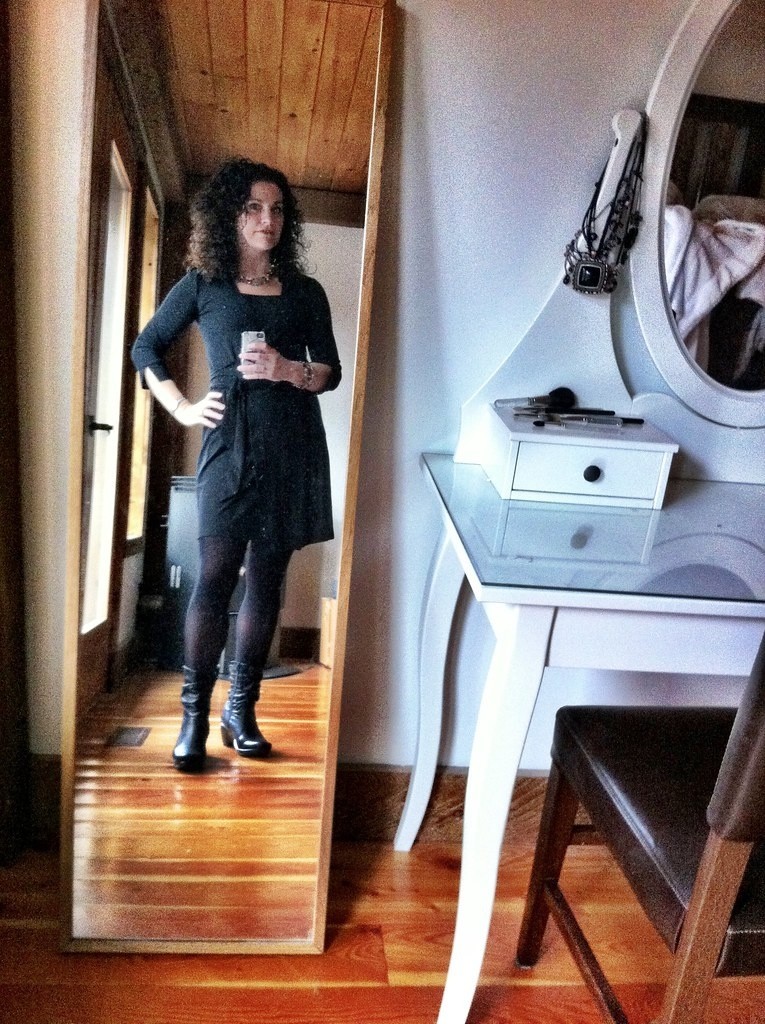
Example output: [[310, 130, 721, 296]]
[[494, 387, 644, 435]]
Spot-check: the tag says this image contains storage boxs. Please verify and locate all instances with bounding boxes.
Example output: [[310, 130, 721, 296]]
[[466, 471, 658, 568], [486, 400, 680, 510]]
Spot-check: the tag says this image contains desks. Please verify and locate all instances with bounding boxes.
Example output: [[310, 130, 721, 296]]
[[392, 452, 765, 1024]]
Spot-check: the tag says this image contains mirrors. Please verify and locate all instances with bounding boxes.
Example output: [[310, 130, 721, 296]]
[[629, 0, 765, 429], [56, 0, 399, 955]]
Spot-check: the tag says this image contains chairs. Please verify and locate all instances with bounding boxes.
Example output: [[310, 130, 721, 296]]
[[515, 624, 765, 1024]]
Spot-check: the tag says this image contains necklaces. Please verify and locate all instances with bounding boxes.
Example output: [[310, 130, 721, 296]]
[[236, 257, 282, 287], [563, 112, 649, 295]]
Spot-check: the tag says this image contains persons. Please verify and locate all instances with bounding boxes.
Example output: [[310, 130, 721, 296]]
[[131, 162, 342, 771]]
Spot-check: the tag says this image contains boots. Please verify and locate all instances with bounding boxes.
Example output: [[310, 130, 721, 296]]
[[172, 665, 220, 770], [220, 660, 272, 757]]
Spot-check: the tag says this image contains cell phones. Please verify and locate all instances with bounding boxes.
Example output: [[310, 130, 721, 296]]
[[242, 331, 265, 374]]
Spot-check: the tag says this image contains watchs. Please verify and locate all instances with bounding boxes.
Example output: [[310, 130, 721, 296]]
[[295, 360, 316, 390]]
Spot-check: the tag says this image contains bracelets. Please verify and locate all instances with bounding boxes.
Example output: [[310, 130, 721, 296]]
[[171, 394, 186, 418]]
